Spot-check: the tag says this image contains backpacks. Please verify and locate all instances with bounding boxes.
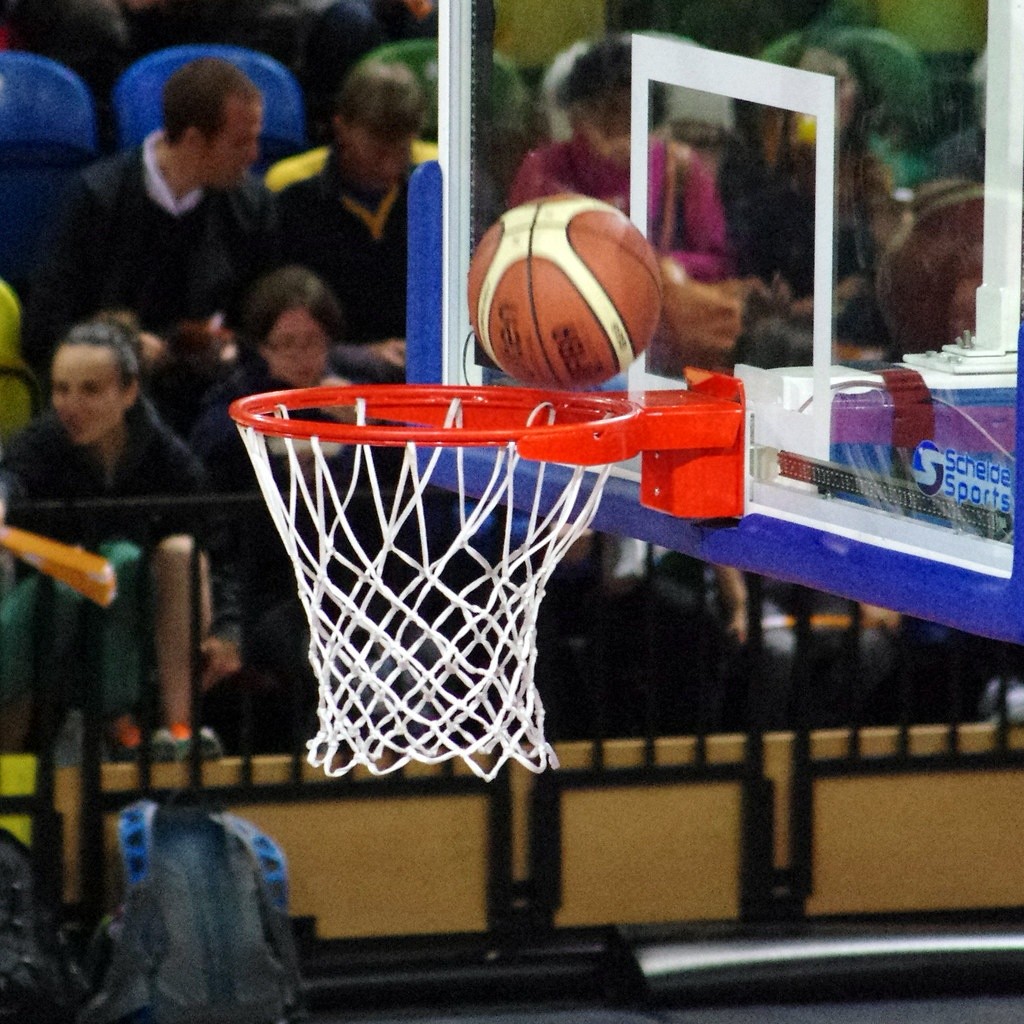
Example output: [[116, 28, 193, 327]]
[[76, 781, 305, 1024]]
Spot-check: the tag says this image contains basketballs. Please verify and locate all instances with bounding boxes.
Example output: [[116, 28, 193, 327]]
[[467, 194, 660, 389]]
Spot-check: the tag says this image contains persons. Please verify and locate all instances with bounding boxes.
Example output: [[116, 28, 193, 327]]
[[0, 0, 1024, 763]]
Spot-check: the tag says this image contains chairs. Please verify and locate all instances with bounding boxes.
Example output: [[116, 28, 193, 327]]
[[0, 27, 935, 286]]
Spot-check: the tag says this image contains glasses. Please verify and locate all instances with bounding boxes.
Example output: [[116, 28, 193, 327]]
[[263, 337, 326, 359]]
